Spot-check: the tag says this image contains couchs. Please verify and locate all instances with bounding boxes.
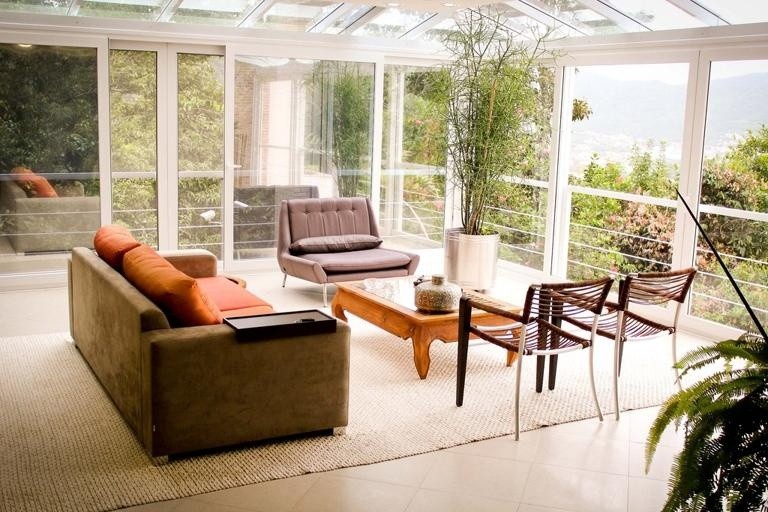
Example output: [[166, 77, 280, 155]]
[[1, 170, 102, 262], [272, 195, 422, 310], [66, 236, 351, 469], [200, 182, 324, 260]]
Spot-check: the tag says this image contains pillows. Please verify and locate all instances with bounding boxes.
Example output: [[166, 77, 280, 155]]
[[120, 242, 227, 327], [91, 222, 142, 275], [287, 232, 385, 257], [8, 166, 58, 198]]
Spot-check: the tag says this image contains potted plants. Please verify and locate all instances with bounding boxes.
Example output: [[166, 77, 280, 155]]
[[408, 1, 583, 297]]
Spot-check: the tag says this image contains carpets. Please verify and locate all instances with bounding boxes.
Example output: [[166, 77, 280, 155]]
[[0, 295, 763, 512]]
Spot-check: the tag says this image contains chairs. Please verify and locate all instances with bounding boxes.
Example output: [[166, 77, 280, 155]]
[[547, 263, 701, 422], [453, 275, 616, 444]]
